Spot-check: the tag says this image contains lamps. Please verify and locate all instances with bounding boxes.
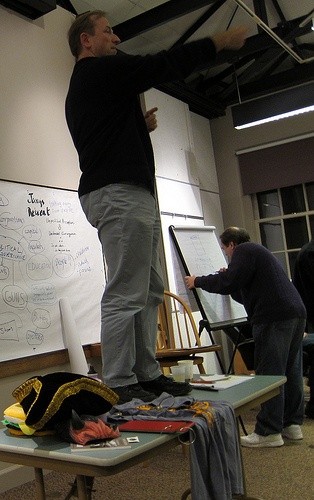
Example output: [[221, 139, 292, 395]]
[[231, 62, 314, 131]]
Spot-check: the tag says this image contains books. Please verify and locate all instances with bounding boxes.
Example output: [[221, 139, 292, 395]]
[[70, 437, 131, 452]]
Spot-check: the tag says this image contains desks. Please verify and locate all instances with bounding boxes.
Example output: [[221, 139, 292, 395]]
[[0, 373, 288, 500]]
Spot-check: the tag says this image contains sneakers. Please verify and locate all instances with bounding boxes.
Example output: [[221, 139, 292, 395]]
[[139, 375, 193, 397], [111, 384, 157, 405], [282, 424, 303, 440], [240, 431, 284, 448]]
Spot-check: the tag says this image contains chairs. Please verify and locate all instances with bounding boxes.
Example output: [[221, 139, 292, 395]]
[[155, 291, 223, 459]]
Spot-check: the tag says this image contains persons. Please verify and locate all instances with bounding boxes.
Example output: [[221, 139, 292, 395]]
[[292, 238, 314, 421], [183, 226, 306, 447], [64, 10, 247, 404]]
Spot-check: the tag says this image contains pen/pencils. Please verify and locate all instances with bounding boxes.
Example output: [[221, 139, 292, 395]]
[[216, 271, 219, 272], [191, 380, 214, 384]]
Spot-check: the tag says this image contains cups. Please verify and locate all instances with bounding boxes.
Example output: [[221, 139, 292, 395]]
[[177, 360, 195, 380], [170, 365, 185, 383]]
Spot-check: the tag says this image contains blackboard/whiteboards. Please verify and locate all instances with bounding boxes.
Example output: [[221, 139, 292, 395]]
[[170, 223, 250, 326], [1, 178, 109, 363]]
